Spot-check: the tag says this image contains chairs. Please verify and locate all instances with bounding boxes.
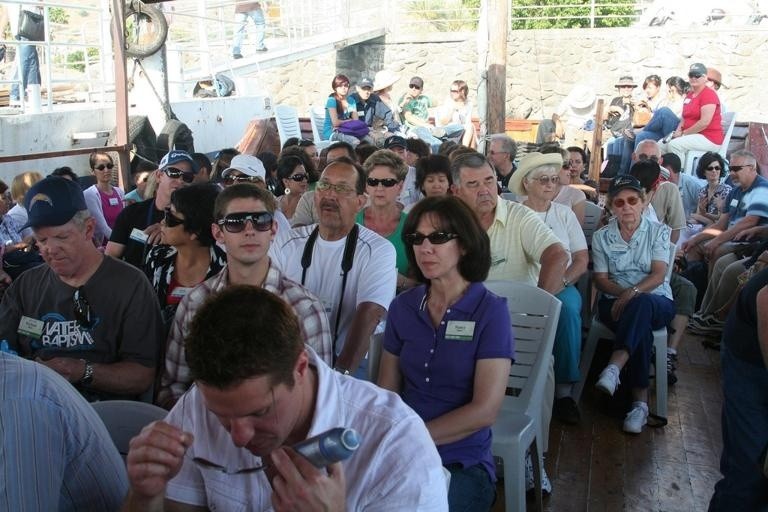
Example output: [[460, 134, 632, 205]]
[[684, 110, 735, 175], [274, 104, 330, 152], [577, 243, 676, 419], [90, 400, 169, 463], [308, 105, 338, 145], [369, 281, 562, 512], [580, 201, 607, 248]]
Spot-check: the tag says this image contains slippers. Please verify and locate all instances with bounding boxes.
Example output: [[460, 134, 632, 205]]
[[621, 129, 635, 142]]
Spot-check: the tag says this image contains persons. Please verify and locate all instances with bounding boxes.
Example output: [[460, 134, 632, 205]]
[[1, 64, 768, 512], [232, 0, 268, 59], [0, 0, 52, 114]]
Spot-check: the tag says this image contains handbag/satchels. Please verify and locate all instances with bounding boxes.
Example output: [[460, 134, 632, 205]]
[[338, 120, 369, 137], [3, 245, 42, 271], [632, 99, 651, 126]]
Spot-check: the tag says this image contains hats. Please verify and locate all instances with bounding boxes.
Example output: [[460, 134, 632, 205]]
[[357, 77, 373, 87], [158, 149, 194, 172], [221, 154, 266, 184], [373, 69, 402, 91], [607, 160, 671, 200], [383, 136, 406, 149], [410, 77, 422, 87], [18, 176, 87, 234], [688, 63, 729, 90], [507, 152, 562, 196], [614, 76, 637, 87]]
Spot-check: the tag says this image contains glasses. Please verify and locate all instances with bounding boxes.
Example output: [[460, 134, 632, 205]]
[[706, 165, 751, 170], [216, 210, 273, 234], [180, 384, 278, 475], [160, 206, 185, 228], [73, 286, 82, 317], [639, 154, 658, 163], [338, 83, 350, 87], [561, 161, 569, 169], [286, 173, 398, 193], [94, 163, 113, 170], [532, 175, 558, 184], [410, 85, 420, 90], [162, 168, 272, 186], [614, 197, 637, 207], [404, 232, 459, 245]]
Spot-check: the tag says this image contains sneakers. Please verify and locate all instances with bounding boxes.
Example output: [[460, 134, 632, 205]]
[[651, 349, 677, 384], [554, 395, 578, 423], [595, 364, 619, 396], [622, 401, 648, 433], [687, 311, 726, 337], [525, 454, 551, 497]]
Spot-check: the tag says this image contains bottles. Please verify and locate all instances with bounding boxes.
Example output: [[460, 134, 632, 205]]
[[289, 425, 361, 469]]
